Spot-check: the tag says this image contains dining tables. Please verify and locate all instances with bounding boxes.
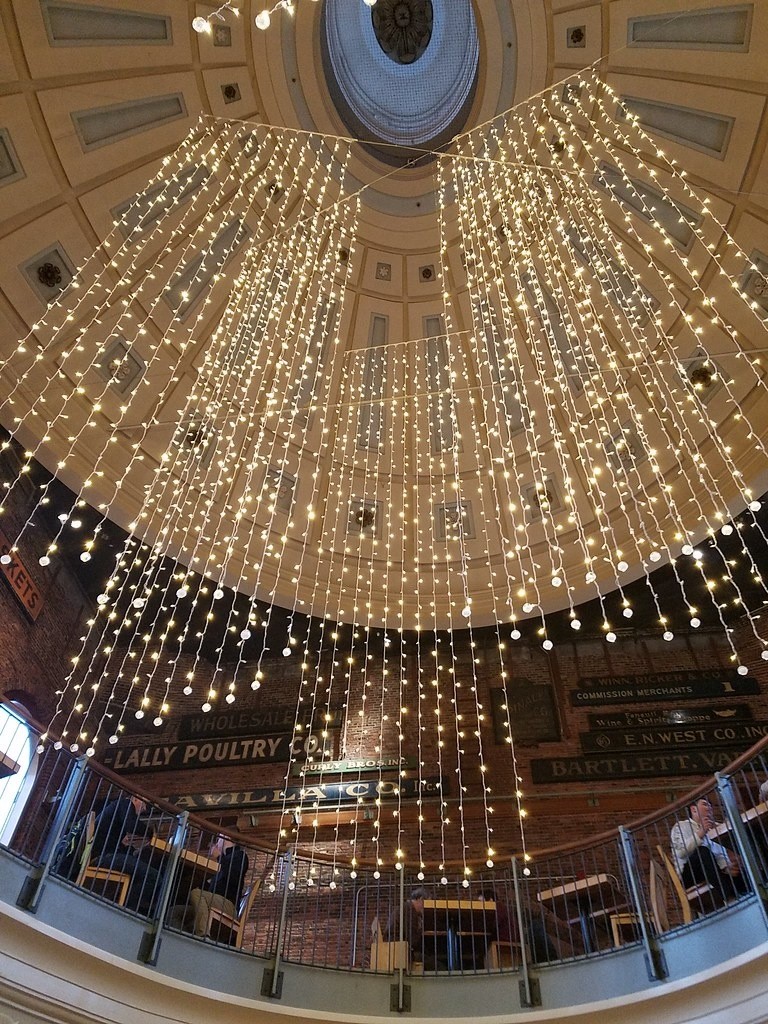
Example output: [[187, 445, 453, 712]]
[[537, 874, 629, 955], [424, 899, 496, 971], [707, 799, 768, 867], [152, 835, 222, 908]]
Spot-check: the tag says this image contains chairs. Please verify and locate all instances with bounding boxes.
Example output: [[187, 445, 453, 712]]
[[609, 859, 670, 948], [657, 845, 723, 924], [482, 925, 531, 971], [206, 879, 261, 948], [370, 917, 409, 973], [76, 811, 131, 907]]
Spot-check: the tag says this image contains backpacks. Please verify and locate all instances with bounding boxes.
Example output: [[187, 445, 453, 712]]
[[57, 815, 84, 865]]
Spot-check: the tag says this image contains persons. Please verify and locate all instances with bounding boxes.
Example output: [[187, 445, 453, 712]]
[[168, 825, 249, 941], [383, 887, 444, 972], [670, 798, 739, 914], [475, 888, 518, 948], [89, 786, 162, 915]]
